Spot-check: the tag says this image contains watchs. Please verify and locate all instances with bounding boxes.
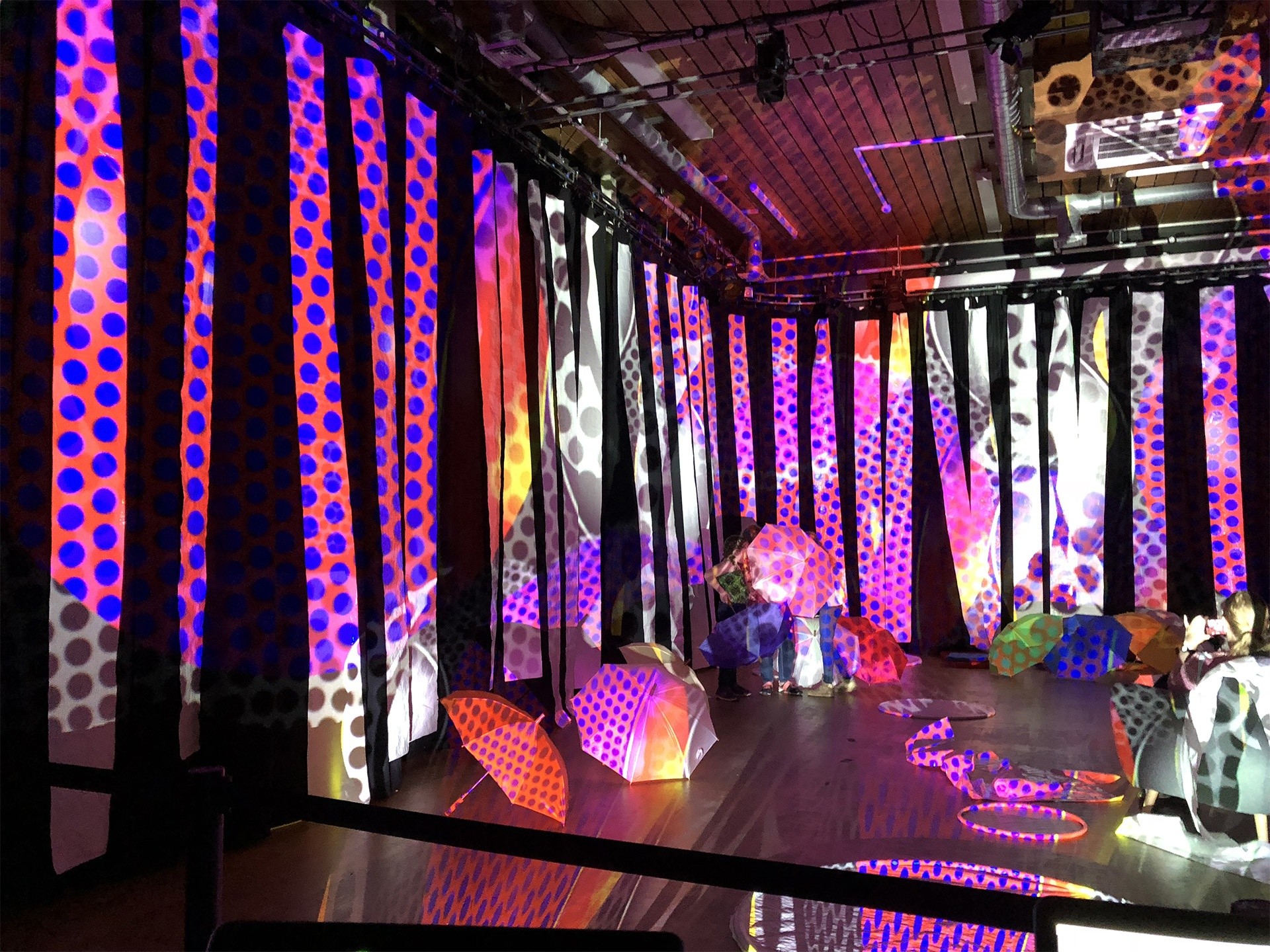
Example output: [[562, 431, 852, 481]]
[[1181, 645, 1195, 654]]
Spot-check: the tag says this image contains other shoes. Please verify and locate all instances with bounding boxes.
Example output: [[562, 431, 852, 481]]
[[778, 680, 803, 696], [807, 680, 835, 697], [752, 667, 761, 676], [759, 681, 773, 696], [714, 684, 752, 701], [834, 675, 858, 693]]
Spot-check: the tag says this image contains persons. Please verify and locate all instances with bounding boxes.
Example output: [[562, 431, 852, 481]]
[[704, 536, 756, 701], [737, 524, 804, 696], [806, 531, 858, 697], [1127, 589, 1270, 842]]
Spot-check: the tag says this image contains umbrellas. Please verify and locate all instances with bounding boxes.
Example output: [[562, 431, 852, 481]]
[[569, 663, 719, 786], [746, 523, 836, 619], [1114, 609, 1185, 674], [837, 617, 909, 687], [618, 643, 709, 712], [1042, 614, 1135, 682], [772, 617, 863, 689], [696, 599, 794, 669], [437, 690, 570, 827], [988, 613, 1064, 678]]
